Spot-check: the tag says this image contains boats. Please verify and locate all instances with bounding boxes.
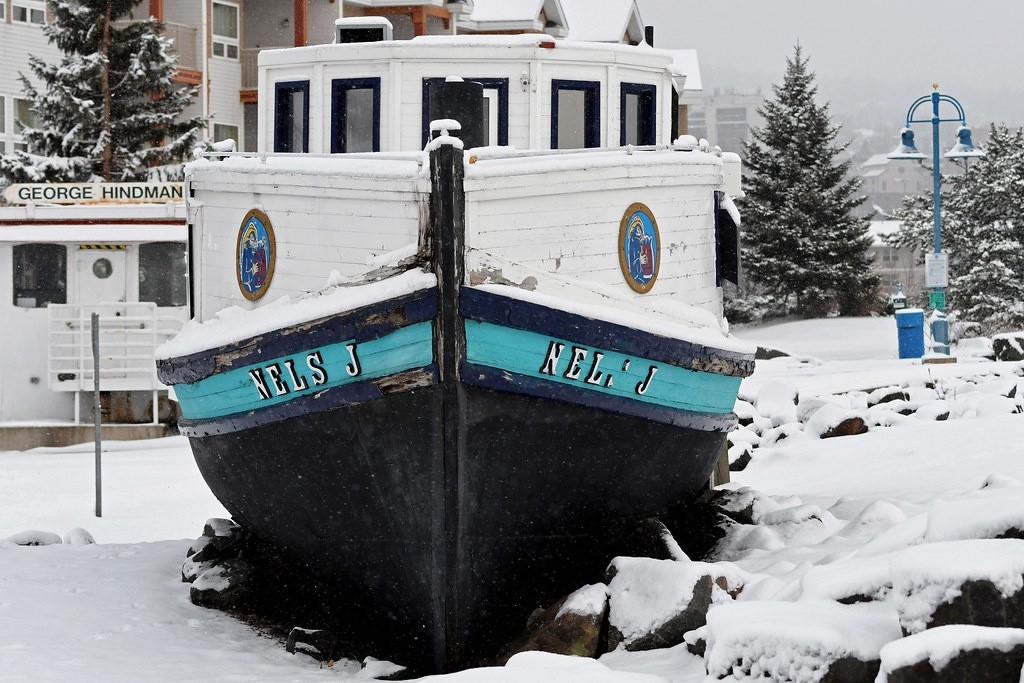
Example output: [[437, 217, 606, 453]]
[[156, 19, 754, 679]]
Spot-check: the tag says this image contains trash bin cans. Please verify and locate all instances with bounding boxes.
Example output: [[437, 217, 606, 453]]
[[896, 313, 925, 359]]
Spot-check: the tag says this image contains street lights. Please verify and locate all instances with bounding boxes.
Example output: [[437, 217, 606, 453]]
[[886, 82, 986, 355]]
